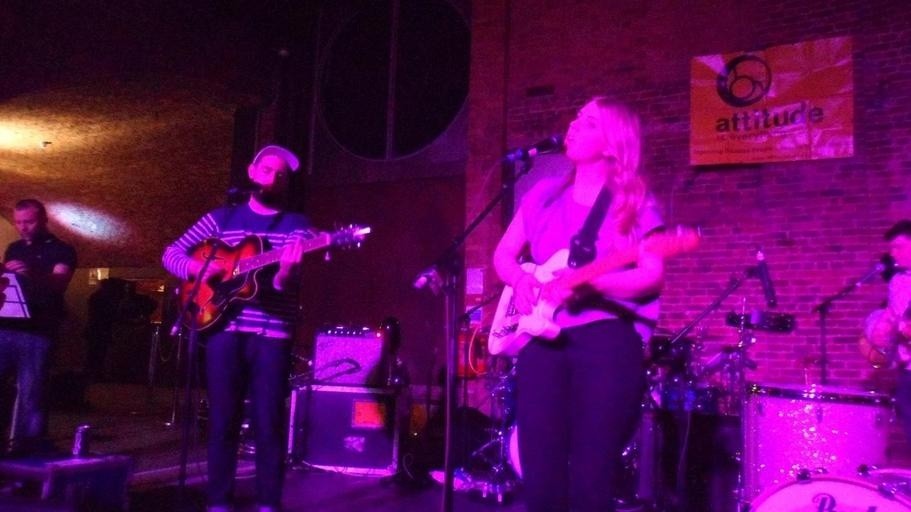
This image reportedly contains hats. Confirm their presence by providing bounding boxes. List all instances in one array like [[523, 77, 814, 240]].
[[251, 146, 301, 173]]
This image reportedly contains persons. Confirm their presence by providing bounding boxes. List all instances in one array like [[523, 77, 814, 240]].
[[490, 91, 667, 511], [857, 220, 911, 464], [0, 196, 77, 449], [160, 143, 321, 512]]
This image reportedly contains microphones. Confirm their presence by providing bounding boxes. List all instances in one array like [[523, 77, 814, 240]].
[[504, 134, 563, 163], [227, 184, 262, 200], [755, 247, 778, 307]]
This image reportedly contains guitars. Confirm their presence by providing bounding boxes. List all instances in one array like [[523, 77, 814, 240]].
[[178, 226, 374, 334], [862, 294, 909, 366], [489, 229, 692, 357]]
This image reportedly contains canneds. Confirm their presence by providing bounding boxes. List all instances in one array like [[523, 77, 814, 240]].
[[70, 424, 91, 456]]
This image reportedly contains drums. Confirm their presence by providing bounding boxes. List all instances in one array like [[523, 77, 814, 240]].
[[457, 327, 512, 383], [749, 466, 911, 510], [622, 406, 712, 507], [504, 423, 521, 477], [741, 379, 899, 509]]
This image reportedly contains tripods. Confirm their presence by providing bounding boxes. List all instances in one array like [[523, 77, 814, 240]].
[[455, 373, 516, 504]]
